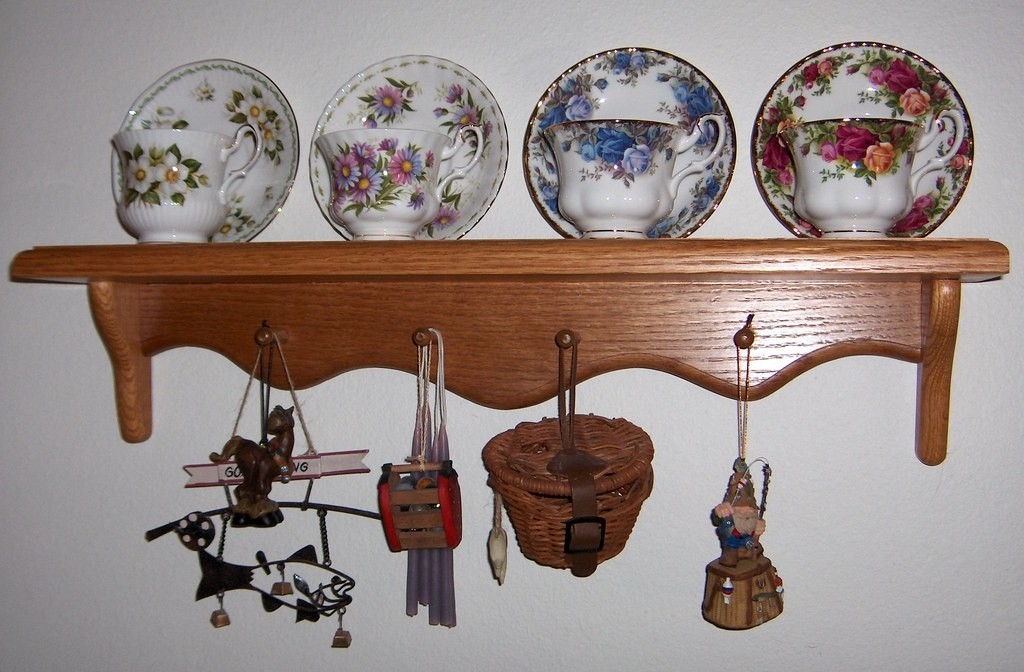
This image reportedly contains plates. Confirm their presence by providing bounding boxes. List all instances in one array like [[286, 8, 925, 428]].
[[308, 54, 509, 243], [748, 41, 973, 240], [522, 47, 736, 241], [110, 58, 300, 244]]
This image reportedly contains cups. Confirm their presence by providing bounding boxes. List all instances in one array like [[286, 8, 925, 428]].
[[314, 124, 483, 241], [544, 113, 726, 238], [110, 120, 262, 244], [779, 109, 964, 240]]
